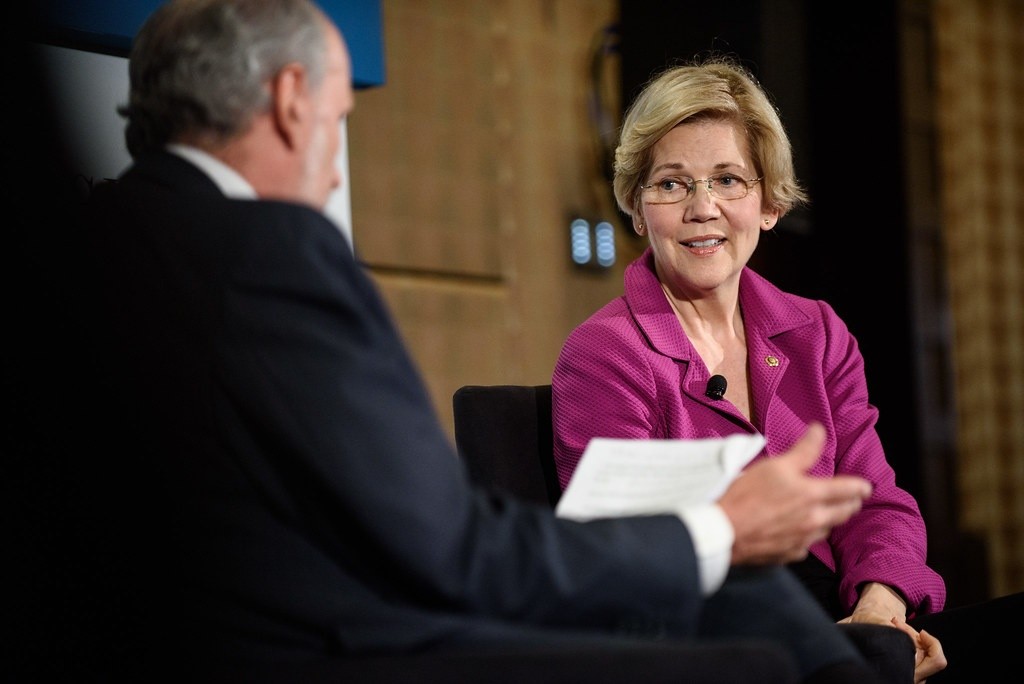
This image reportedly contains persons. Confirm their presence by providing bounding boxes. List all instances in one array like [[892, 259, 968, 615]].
[[0, 0, 874, 684], [549, 64, 950, 684]]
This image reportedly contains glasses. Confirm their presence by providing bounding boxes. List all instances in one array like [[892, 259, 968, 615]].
[[641, 173, 766, 205]]
